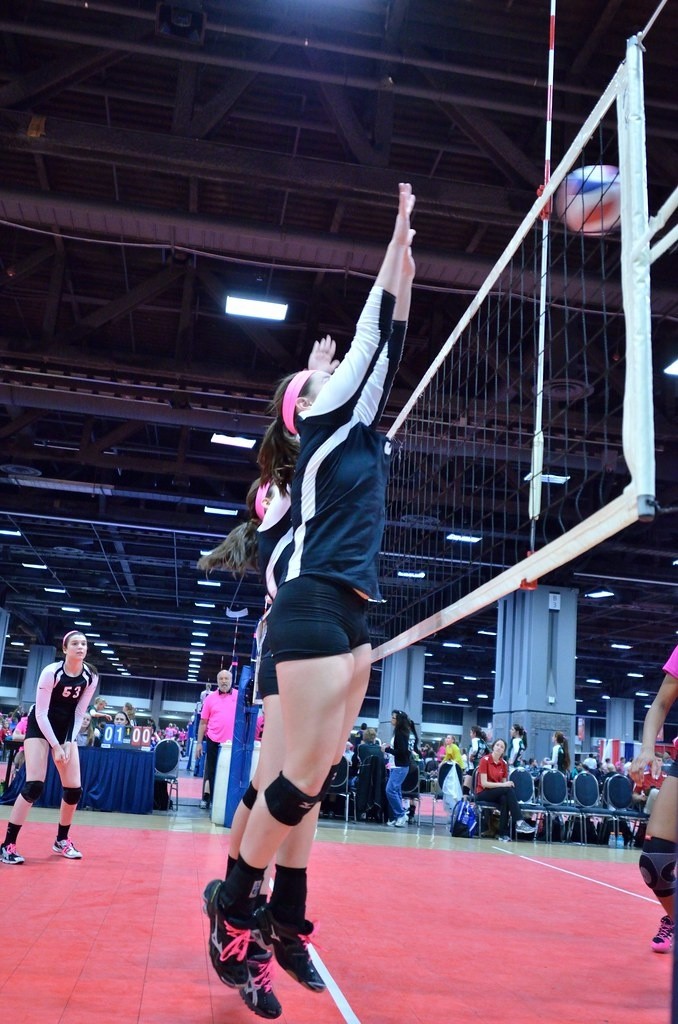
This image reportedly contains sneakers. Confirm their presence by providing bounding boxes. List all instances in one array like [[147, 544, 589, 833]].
[[205, 879, 251, 987], [396, 814, 409, 826], [499, 835, 512, 842], [515, 821, 535, 834], [53, 837, 83, 859], [238, 952, 283, 1020], [388, 820, 396, 827], [252, 905, 327, 993], [0, 843, 26, 865], [651, 915, 676, 952]]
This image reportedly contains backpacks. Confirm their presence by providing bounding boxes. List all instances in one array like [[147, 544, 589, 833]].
[[451, 797, 478, 836]]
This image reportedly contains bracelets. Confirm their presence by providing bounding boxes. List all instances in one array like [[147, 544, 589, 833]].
[[197, 741, 203, 745]]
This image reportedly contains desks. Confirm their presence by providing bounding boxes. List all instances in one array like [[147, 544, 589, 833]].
[[0, 746, 154, 815]]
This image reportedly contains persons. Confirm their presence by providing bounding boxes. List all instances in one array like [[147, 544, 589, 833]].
[[470, 723, 597, 842], [75, 697, 186, 756], [627, 643, 678, 952], [600, 751, 674, 801], [0, 630, 99, 862], [204, 181, 415, 1019], [194, 670, 264, 808], [343, 710, 462, 826], [0, 712, 28, 771]]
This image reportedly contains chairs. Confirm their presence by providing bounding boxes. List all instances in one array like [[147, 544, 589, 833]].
[[470, 766, 650, 845], [153, 738, 181, 811], [321, 749, 425, 826]]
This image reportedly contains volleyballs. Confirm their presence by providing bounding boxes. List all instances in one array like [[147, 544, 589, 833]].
[[556, 165, 621, 236]]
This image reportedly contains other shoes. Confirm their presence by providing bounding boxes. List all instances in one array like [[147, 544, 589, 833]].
[[199, 799, 209, 808]]
